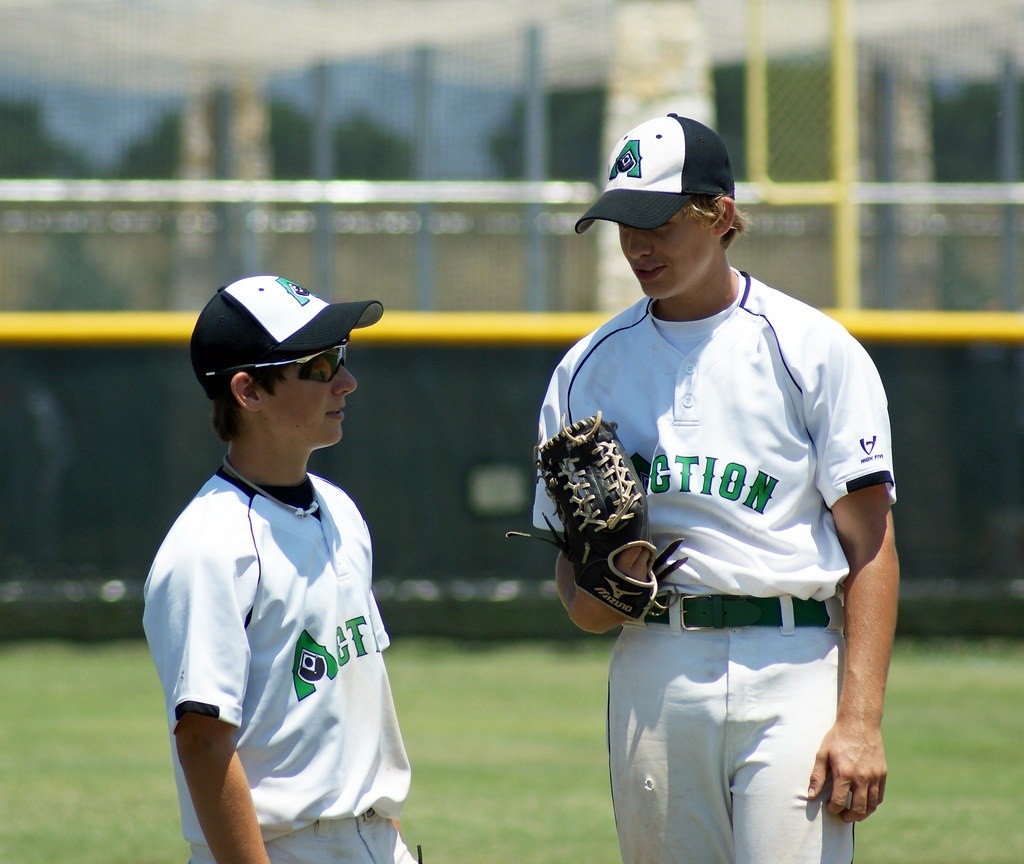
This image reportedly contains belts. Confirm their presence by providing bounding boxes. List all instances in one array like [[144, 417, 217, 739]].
[[644, 593, 830, 629]]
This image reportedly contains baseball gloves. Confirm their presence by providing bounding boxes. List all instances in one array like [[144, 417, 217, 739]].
[[536, 416, 659, 623]]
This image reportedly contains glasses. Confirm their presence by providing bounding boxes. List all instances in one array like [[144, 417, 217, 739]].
[[202, 340, 352, 381]]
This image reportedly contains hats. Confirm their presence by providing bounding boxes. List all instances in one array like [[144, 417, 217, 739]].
[[191, 274, 385, 399], [575, 112, 735, 235]]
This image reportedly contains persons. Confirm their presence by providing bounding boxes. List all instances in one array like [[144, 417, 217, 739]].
[[142, 277, 432, 864], [533, 112, 902, 864]]
